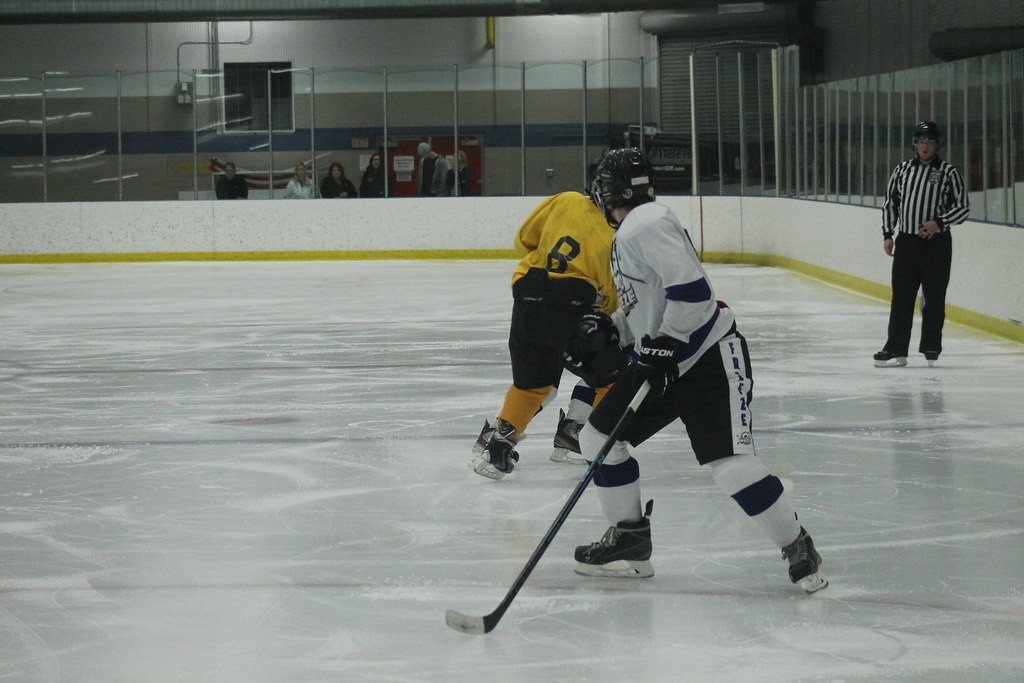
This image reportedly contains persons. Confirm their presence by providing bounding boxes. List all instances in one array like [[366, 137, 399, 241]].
[[445, 150, 470, 197], [575, 148, 823, 583], [873, 123, 971, 360], [283, 163, 322, 199], [479, 192, 621, 474], [215, 162, 249, 201], [321, 162, 358, 199], [361, 153, 395, 197], [417, 142, 448, 197]]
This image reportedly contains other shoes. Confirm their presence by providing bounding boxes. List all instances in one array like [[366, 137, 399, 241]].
[[873, 349, 908, 367], [924, 350, 938, 367]]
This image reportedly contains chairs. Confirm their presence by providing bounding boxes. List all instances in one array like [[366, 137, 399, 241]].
[[178, 188, 289, 200]]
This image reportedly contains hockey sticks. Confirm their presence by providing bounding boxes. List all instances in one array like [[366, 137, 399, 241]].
[[444, 375, 654, 636]]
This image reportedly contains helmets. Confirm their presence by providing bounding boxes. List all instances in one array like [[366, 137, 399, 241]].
[[911, 121, 942, 151], [593, 147, 654, 208]]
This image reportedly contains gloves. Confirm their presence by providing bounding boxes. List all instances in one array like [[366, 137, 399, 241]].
[[626, 334, 680, 405], [565, 312, 623, 386]]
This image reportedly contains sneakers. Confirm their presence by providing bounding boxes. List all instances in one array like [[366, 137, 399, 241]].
[[779, 512, 828, 593], [472, 419, 519, 466], [573, 499, 655, 578], [473, 416, 517, 479], [550, 408, 589, 464]]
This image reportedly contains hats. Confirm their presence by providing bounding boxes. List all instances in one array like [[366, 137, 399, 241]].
[[418, 142, 431, 155]]
